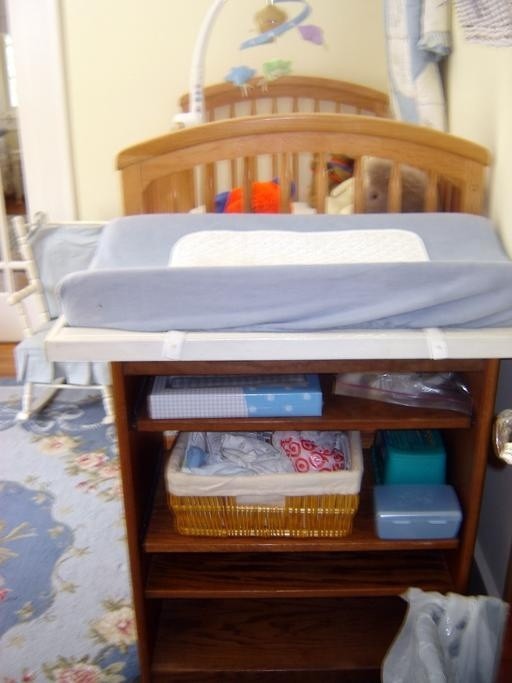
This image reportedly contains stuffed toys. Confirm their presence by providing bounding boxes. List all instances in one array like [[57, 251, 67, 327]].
[[308, 153, 444, 214], [215, 177, 296, 213]]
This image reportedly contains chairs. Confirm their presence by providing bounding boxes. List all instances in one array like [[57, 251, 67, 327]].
[[2, 215, 116, 426]]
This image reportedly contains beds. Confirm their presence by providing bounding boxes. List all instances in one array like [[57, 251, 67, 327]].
[[117, 71, 495, 217]]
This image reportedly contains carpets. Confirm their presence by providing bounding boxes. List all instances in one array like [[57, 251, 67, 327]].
[[2, 375, 512, 682]]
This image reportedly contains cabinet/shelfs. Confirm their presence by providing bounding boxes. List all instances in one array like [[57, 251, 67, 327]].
[[114, 359, 504, 682]]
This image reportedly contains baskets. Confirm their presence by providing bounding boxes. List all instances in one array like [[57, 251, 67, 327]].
[[163, 429, 366, 540]]
